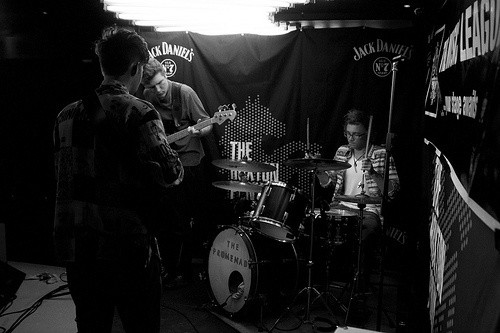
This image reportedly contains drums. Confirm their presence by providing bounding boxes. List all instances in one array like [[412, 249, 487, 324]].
[[328, 209, 358, 245], [208, 226, 300, 314], [252, 181, 310, 242]]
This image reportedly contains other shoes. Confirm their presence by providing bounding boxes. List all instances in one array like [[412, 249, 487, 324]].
[[165, 274, 194, 291]]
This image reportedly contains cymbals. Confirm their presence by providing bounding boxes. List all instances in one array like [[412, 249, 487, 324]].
[[284, 155, 352, 172], [333, 193, 390, 205], [212, 179, 266, 192], [212, 158, 278, 172]]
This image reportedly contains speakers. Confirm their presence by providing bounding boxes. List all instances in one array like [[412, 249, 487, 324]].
[[0, 261, 26, 309]]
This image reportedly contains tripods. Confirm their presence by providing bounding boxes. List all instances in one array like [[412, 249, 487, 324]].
[[267, 166, 340, 333]]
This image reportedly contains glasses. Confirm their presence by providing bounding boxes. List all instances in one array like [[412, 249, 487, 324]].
[[343, 130, 367, 140]]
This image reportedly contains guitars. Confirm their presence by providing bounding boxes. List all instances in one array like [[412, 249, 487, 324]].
[[167, 103, 237, 145]]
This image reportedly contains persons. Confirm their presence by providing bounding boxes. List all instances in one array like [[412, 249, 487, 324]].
[[136, 56, 212, 292], [52, 21, 184, 333], [305, 109, 400, 314]]
